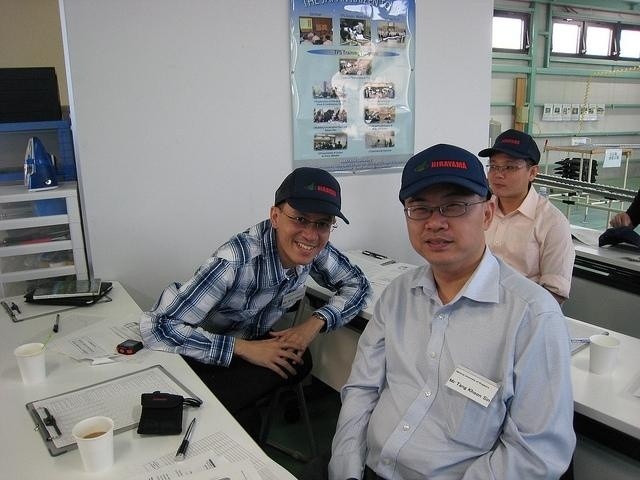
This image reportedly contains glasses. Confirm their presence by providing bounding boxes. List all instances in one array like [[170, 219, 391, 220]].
[[403, 201, 486, 220], [485, 163, 530, 173], [279, 207, 333, 233]]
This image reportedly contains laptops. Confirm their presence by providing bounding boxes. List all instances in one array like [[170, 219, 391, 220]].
[[22, 283, 114, 305]]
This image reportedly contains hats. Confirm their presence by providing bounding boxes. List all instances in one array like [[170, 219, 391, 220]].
[[274, 166, 350, 226], [398, 142, 489, 200], [478, 128, 541, 167]]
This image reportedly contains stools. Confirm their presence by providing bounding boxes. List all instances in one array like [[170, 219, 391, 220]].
[[234, 332, 323, 480]]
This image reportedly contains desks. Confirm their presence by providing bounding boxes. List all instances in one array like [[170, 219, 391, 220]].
[[544, 145, 633, 228], [299, 248, 639, 480], [559, 224, 639, 339], [0, 280, 295, 480]]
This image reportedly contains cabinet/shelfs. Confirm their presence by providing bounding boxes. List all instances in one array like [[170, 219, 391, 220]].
[[1, 121, 93, 300]]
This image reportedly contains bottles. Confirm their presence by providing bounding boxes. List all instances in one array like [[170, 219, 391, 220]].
[[539, 186, 546, 197]]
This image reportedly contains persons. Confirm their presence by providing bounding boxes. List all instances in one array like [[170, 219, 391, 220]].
[[327, 144, 578, 480], [608, 185, 640, 230], [138, 167, 371, 411], [479, 128, 576, 306]]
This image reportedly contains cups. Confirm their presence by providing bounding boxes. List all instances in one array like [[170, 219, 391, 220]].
[[72, 416, 114, 474], [14, 342, 47, 386], [588, 335, 621, 377]]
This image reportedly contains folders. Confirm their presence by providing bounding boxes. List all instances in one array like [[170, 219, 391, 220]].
[[26, 364, 203, 458], [0, 294, 113, 323]]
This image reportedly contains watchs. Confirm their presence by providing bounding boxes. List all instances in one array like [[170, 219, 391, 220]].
[[312, 312, 327, 331]]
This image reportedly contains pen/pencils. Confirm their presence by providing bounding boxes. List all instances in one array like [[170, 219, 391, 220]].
[[53, 314, 60, 332], [571, 339, 591, 343], [175, 418, 196, 461]]
